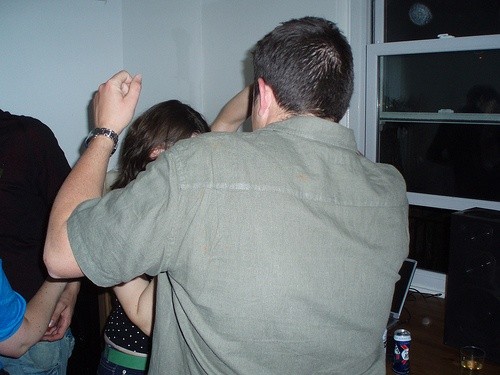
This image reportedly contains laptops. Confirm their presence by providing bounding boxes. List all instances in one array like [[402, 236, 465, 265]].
[[387, 258, 418, 326]]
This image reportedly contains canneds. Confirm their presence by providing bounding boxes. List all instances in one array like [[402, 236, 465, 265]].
[[392, 329, 411, 374]]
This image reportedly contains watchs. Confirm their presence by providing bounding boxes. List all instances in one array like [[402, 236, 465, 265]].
[[84, 128, 119, 158]]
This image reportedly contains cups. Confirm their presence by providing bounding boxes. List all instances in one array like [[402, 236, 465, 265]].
[[459, 346, 486, 375]]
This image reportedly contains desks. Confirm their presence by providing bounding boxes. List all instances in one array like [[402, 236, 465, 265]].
[[386, 290, 500, 375]]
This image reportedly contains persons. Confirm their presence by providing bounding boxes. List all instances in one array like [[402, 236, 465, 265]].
[[0, 99, 212, 375], [42, 17, 409, 375]]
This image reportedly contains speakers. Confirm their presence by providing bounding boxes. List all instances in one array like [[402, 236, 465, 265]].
[[443, 207, 500, 366]]
